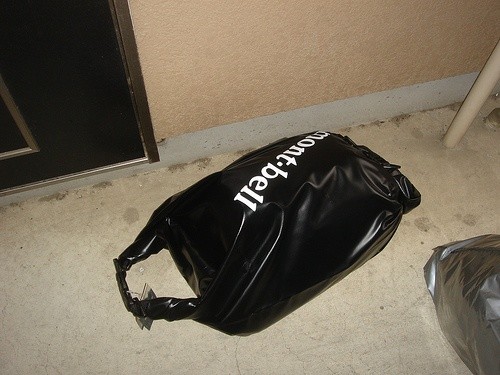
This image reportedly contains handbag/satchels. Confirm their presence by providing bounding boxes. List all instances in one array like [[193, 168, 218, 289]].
[[114, 131, 422, 336], [423, 234, 499, 375]]
